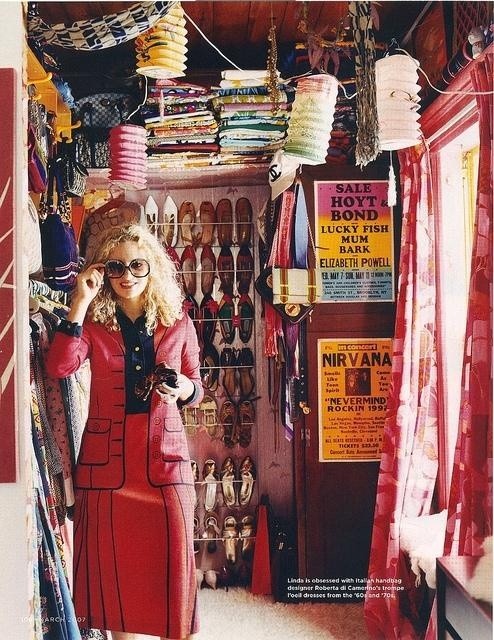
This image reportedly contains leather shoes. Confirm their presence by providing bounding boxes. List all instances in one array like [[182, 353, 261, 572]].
[[201, 245, 216, 294], [181, 294, 254, 344], [218, 248, 234, 295], [163, 196, 178, 247], [144, 195, 158, 240], [236, 247, 253, 294], [180, 245, 196, 296]]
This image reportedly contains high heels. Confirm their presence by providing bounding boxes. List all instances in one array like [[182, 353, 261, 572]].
[[205, 570, 217, 590], [239, 565, 249, 589], [196, 568, 203, 590], [222, 567, 233, 592]]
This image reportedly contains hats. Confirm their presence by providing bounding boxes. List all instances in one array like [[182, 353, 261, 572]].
[[268, 149, 301, 202]]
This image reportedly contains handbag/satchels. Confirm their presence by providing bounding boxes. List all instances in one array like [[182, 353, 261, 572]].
[[271, 181, 323, 307], [72, 128, 111, 168], [271, 518, 297, 604], [73, 89, 140, 129], [254, 181, 317, 325], [27, 84, 88, 291]]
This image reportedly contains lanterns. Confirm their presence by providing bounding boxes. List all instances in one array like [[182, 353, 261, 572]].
[[373, 54, 421, 153], [108, 123, 149, 192], [133, 1, 188, 80], [280, 73, 340, 165]]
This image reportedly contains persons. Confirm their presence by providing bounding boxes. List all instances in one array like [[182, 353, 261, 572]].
[[44, 220, 206, 640]]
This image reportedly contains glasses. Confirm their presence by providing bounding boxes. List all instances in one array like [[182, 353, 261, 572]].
[[134, 361, 177, 407], [105, 258, 150, 279]]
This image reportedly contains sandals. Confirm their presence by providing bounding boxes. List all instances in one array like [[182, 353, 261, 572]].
[[190, 455, 256, 565], [216, 199, 232, 247], [197, 201, 216, 248], [181, 344, 255, 448], [179, 200, 199, 247], [235, 198, 252, 247]]
